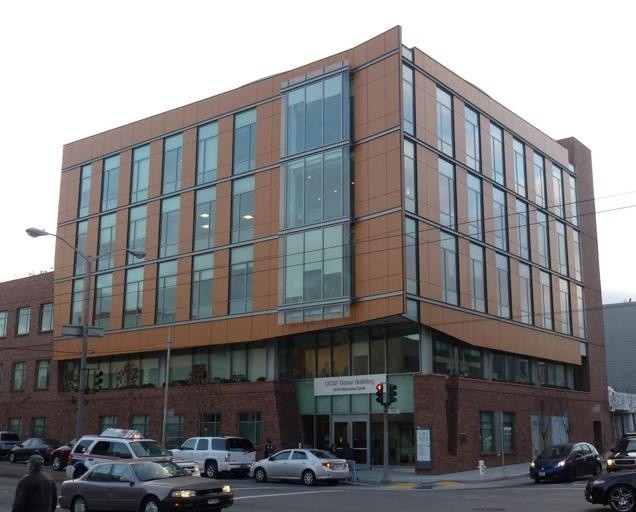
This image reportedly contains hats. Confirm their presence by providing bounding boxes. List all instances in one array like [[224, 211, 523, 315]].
[[25, 455, 44, 464]]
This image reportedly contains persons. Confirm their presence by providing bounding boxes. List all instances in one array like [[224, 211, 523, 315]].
[[341, 442, 357, 481], [263, 438, 273, 457], [11, 455, 58, 512], [329, 442, 339, 457]]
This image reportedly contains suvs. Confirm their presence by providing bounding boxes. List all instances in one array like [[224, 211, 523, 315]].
[[64, 428, 201, 477], [605, 432, 636, 472], [168, 434, 258, 478]]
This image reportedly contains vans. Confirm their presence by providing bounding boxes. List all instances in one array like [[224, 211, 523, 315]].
[[530, 441, 603, 484]]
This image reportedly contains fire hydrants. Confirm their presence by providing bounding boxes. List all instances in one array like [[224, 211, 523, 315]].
[[475, 458, 488, 478]]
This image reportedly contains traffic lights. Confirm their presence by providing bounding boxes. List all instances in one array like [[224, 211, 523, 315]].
[[375, 383, 383, 404], [388, 383, 398, 404]]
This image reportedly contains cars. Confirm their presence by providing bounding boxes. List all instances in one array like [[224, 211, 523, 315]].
[[0, 430, 81, 471], [584, 469, 636, 512], [56, 459, 234, 512], [249, 448, 351, 486]]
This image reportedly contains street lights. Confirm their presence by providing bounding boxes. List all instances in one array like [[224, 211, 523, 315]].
[[23, 228, 146, 437]]
